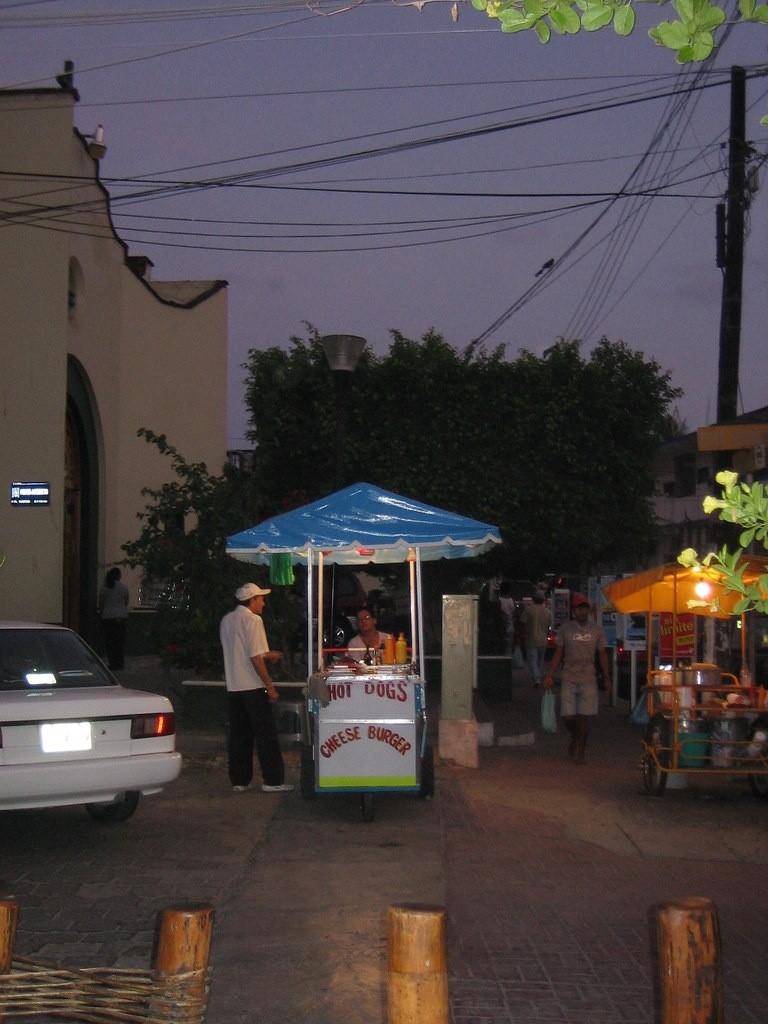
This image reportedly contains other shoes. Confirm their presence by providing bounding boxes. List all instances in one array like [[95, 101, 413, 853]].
[[569, 740, 577, 754], [576, 752, 588, 764], [533, 681, 540, 689], [233, 784, 252, 793], [263, 784, 294, 792]]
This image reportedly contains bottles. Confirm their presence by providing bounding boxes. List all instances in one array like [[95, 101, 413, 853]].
[[384, 634, 396, 663], [395, 632, 407, 664], [364, 644, 372, 665]]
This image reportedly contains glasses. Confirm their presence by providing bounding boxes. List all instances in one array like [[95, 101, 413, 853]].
[[358, 616, 369, 622]]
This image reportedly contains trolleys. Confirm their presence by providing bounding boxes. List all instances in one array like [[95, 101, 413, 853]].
[[225, 479, 501, 824], [601, 553, 768, 799]]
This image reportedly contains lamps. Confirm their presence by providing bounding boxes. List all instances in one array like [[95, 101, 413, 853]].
[[80, 125, 107, 159]]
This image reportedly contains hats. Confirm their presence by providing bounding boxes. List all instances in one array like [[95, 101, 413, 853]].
[[235, 583, 271, 601], [535, 590, 545, 601], [571, 593, 590, 608]]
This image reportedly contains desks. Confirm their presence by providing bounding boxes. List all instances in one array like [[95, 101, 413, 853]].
[[181, 679, 306, 688], [424, 655, 513, 702]]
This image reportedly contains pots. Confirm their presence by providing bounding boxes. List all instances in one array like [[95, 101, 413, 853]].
[[681, 668, 724, 687]]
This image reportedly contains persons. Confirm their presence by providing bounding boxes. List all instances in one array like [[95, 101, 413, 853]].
[[220, 583, 296, 792], [99, 567, 130, 671], [495, 592, 553, 684], [346, 609, 396, 666], [543, 594, 611, 765]]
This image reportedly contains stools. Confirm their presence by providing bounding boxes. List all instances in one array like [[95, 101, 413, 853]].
[[274, 698, 310, 750]]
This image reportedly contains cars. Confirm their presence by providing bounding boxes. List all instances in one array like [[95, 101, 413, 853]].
[[0, 618, 183, 825], [510, 575, 648, 676]]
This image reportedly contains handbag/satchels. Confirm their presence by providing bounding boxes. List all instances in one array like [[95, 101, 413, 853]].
[[512, 644, 524, 669], [541, 688, 558, 735]]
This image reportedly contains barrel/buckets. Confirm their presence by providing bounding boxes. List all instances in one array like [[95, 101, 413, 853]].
[[678, 732, 709, 768]]
